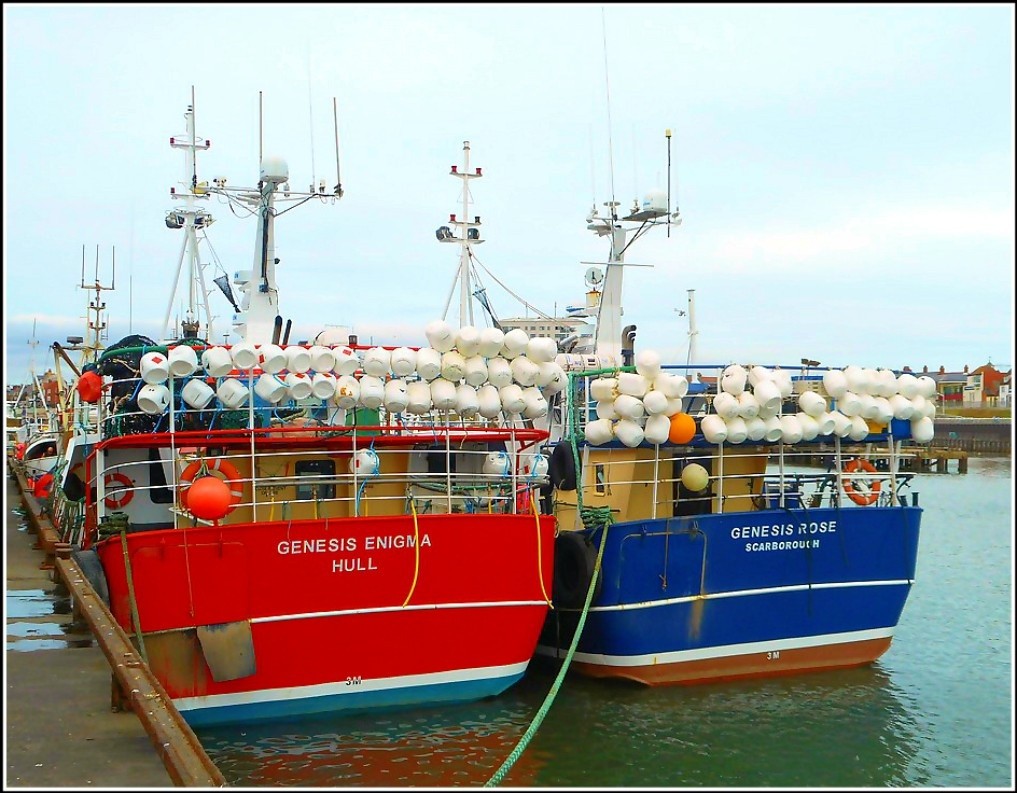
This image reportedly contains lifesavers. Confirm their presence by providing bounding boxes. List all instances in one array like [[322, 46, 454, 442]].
[[517, 485, 529, 515], [542, 441, 582, 495], [554, 530, 603, 607], [177, 459, 244, 520], [842, 459, 881, 505], [104, 473, 134, 509]]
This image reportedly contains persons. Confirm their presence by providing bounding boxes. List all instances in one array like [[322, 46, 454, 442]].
[[41, 446, 53, 457]]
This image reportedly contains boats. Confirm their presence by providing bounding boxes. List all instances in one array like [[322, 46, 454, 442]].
[[427, 128, 942, 688], [14, 84, 579, 727]]
